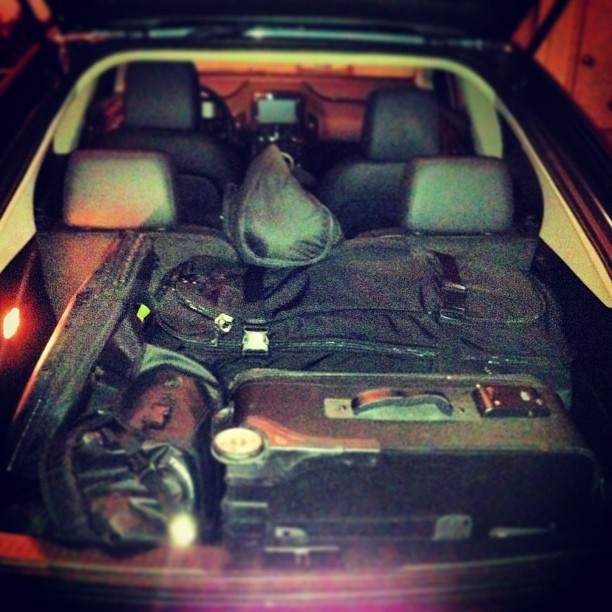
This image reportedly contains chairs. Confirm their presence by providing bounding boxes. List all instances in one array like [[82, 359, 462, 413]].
[[33, 149, 178, 306], [327, 85, 449, 236], [107, 58, 239, 226], [348, 155, 543, 280]]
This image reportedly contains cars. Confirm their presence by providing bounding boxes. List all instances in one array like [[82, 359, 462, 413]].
[[2, 0, 610, 606]]
